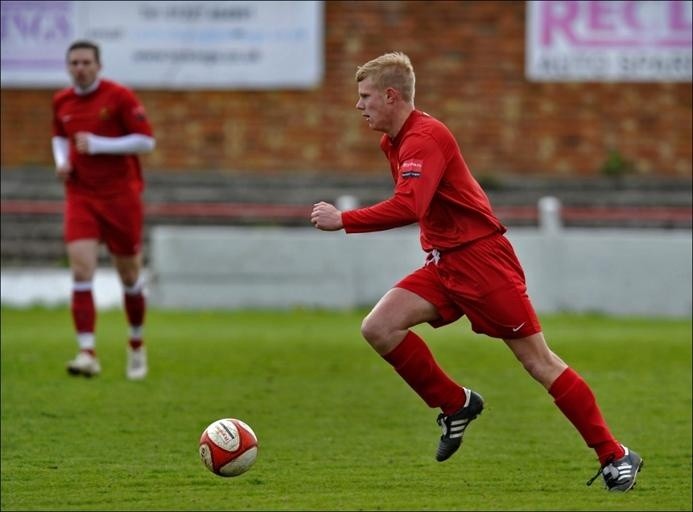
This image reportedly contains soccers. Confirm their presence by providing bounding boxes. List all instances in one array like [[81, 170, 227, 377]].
[[198, 417, 260, 478]]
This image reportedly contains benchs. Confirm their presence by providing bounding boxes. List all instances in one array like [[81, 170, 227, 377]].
[[1, 164, 693, 271]]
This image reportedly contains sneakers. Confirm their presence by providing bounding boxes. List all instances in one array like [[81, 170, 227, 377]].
[[67, 351, 100, 378], [127, 344, 147, 380], [587, 445, 644, 493], [435, 387, 484, 461]]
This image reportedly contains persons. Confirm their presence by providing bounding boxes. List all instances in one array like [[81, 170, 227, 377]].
[[45, 41, 159, 381], [308, 52, 646, 494]]
